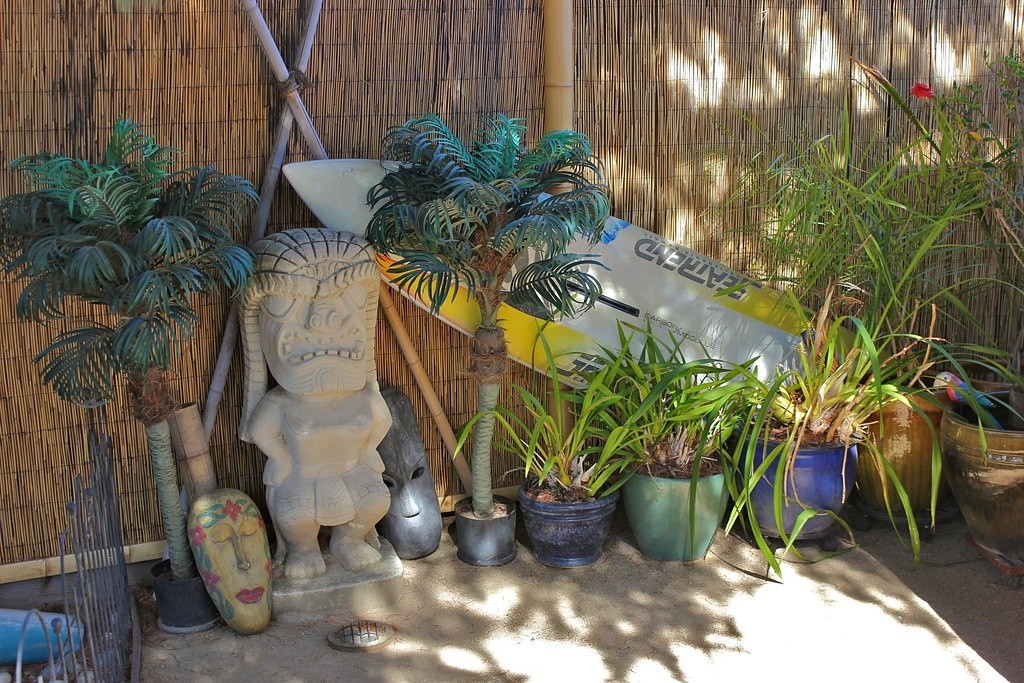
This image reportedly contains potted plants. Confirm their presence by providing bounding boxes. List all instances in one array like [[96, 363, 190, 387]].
[[364, 49, 1024, 589], [0, 114, 261, 633]]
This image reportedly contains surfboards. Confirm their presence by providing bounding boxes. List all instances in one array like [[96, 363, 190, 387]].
[[280, 158, 908, 425]]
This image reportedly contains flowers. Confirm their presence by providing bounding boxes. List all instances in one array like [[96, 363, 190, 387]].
[[910, 82, 934, 99]]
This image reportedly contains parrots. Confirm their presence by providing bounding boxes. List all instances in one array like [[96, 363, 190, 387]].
[[933, 372, 1002, 429]]
